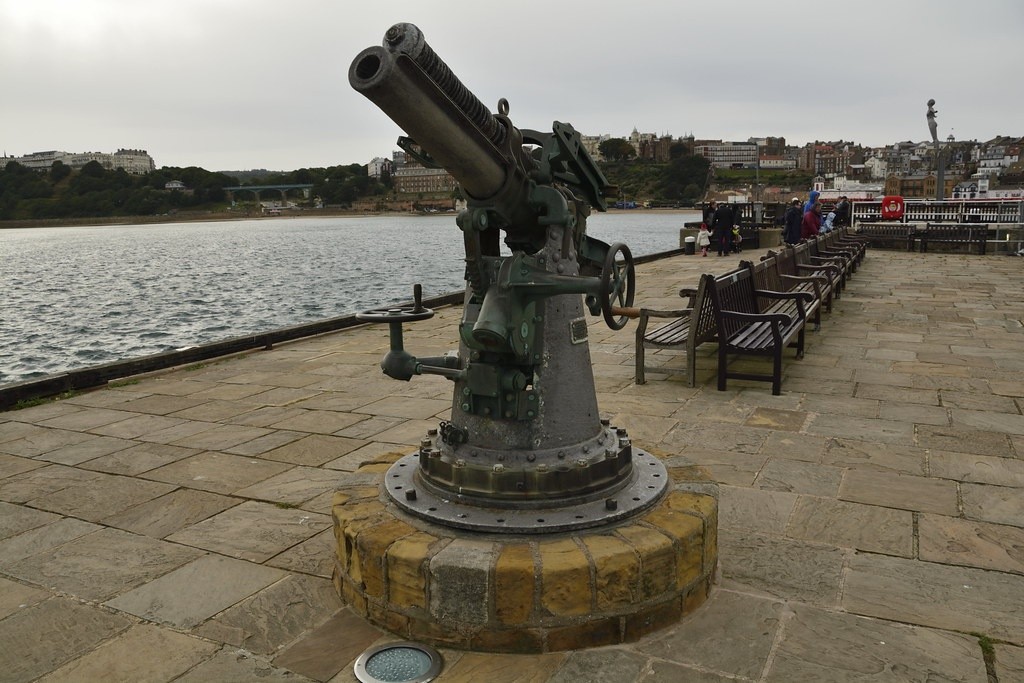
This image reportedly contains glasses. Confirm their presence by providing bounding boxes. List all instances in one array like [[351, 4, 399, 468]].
[[799, 205, 802, 207]]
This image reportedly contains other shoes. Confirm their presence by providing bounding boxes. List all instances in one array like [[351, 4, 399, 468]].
[[717, 254, 721, 257], [702, 254, 707, 257], [724, 254, 730, 256]]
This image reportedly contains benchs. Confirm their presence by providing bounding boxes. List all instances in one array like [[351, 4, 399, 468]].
[[635, 223, 989, 396]]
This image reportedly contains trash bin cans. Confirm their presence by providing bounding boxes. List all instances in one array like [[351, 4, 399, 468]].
[[759, 228, 783, 247]]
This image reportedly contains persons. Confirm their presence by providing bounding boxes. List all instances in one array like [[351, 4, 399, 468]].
[[696, 201, 743, 257], [926, 99, 938, 141], [782, 192, 850, 248]]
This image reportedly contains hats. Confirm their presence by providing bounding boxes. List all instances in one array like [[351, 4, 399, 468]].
[[700, 223, 708, 230], [841, 196, 847, 199]]
